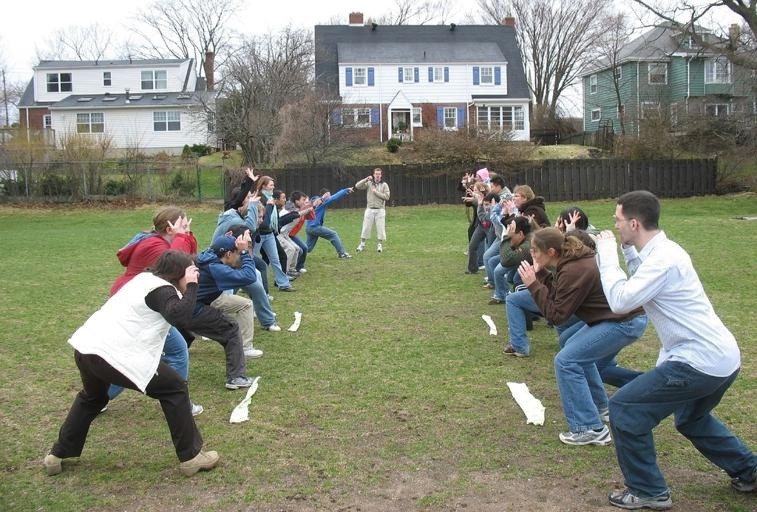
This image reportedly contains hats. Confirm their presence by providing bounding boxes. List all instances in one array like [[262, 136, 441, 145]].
[[213, 236, 236, 251]]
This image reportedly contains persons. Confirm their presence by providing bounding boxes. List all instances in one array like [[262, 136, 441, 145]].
[[456, 165, 646, 445], [97, 165, 355, 421], [37, 248, 220, 477], [586, 188, 756, 511], [355, 162, 390, 255]]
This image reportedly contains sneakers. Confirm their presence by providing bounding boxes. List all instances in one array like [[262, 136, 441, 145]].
[[226, 375, 253, 389], [244, 349, 263, 359], [503, 346, 527, 357], [338, 253, 352, 258], [357, 243, 382, 252], [559, 410, 612, 446], [180, 449, 219, 476], [609, 489, 672, 510], [45, 455, 62, 476], [275, 269, 307, 291], [466, 265, 502, 304], [731, 468, 757, 491], [191, 404, 203, 416], [269, 325, 281, 332]]
